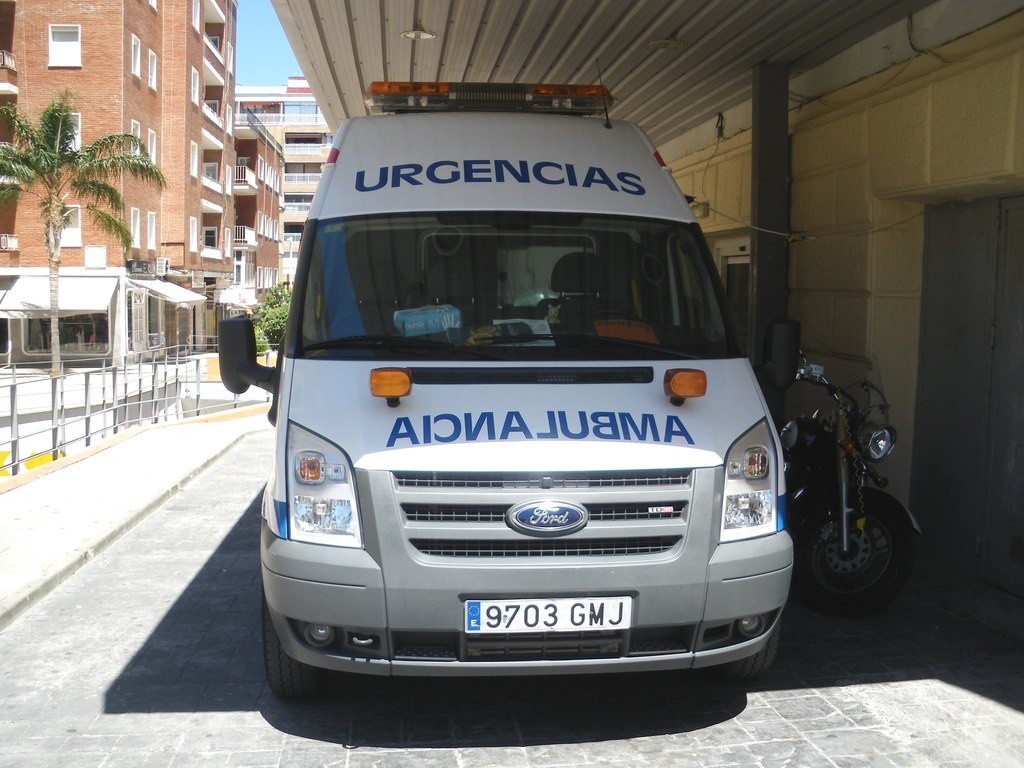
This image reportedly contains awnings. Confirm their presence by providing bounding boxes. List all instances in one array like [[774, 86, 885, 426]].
[[0, 276, 118, 311], [131, 278, 207, 302]]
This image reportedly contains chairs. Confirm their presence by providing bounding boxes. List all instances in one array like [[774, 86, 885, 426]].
[[536, 252, 618, 332]]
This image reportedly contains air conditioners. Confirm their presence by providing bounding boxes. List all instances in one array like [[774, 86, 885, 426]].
[[0, 52, 16, 69], [156, 256, 170, 275], [1, 236, 18, 249]]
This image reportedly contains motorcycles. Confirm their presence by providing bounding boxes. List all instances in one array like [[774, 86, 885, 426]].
[[759, 314, 927, 616]]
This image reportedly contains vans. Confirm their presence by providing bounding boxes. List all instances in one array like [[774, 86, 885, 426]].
[[215, 80, 796, 706]]
